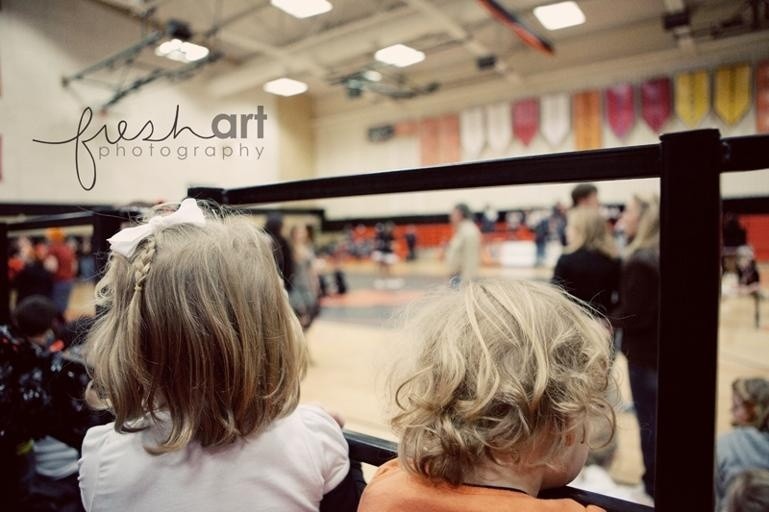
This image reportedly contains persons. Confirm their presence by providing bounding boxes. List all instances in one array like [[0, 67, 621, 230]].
[[0, 180, 768, 332], [603, 188, 659, 498], [346, 275, 615, 512], [0, 293, 114, 512], [719, 467, 768, 510], [713, 372, 768, 507], [66, 194, 366, 512], [549, 206, 624, 383]]
